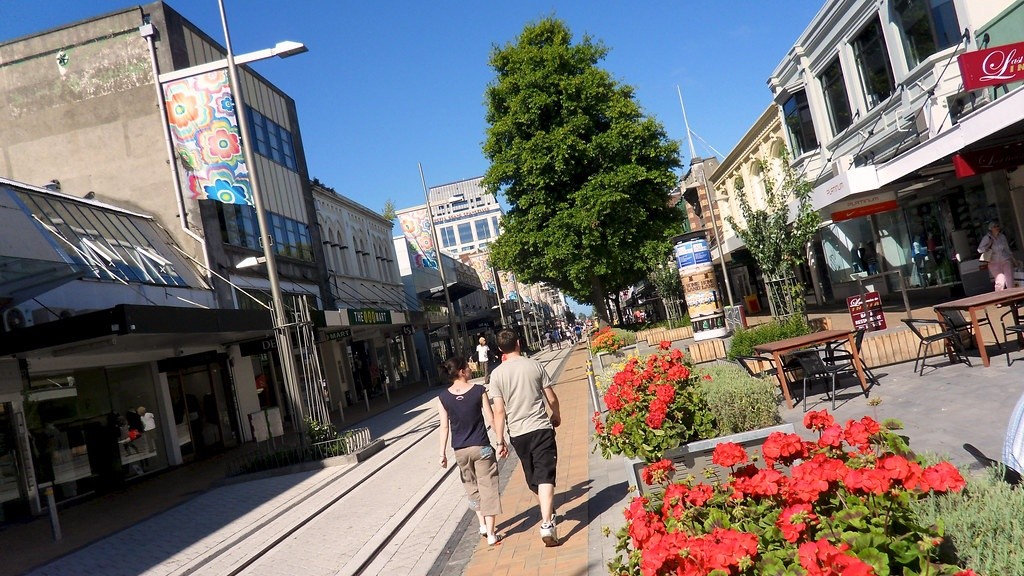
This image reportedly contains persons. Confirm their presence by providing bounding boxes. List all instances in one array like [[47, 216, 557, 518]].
[[117, 416, 139, 457], [437, 357, 509, 546], [138, 407, 156, 453], [545, 328, 562, 352], [565, 324, 581, 343], [851, 239, 877, 274], [912, 233, 939, 286], [490, 328, 562, 547], [594, 317, 598, 328], [635, 309, 646, 322], [978, 221, 1018, 307], [472, 337, 498, 384]]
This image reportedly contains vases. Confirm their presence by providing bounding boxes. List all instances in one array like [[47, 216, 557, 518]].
[[595, 343, 639, 369], [623, 415, 802, 507]]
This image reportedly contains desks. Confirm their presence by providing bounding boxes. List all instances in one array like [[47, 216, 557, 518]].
[[752, 329, 870, 410], [933, 285, 1024, 367]]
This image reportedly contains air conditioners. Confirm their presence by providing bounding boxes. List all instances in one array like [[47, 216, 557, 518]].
[[32, 307, 74, 326], [1, 305, 34, 330]]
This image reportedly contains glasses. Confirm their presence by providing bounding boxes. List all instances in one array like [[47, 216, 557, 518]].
[[992, 225, 1000, 228]]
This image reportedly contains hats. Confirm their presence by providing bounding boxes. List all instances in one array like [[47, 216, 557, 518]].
[[565, 326, 569, 329]]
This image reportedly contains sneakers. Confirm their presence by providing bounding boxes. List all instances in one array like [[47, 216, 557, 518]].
[[540, 522, 559, 547], [550, 514, 556, 527]]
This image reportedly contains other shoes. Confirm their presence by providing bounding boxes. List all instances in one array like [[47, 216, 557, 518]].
[[148, 448, 154, 453], [996, 304, 1001, 307], [479, 525, 489, 535], [487, 535, 503, 544], [125, 453, 132, 457], [134, 452, 139, 454]]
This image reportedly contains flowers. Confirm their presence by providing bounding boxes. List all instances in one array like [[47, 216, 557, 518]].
[[601, 411, 1024, 576], [583, 326, 782, 459]]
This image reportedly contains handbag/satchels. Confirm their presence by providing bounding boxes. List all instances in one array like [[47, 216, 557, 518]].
[[909, 262, 921, 287], [128, 429, 141, 441], [979, 249, 992, 262]]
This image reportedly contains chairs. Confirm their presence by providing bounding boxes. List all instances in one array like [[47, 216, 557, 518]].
[[732, 326, 880, 412], [900, 308, 1002, 377], [1000, 303, 1024, 366]]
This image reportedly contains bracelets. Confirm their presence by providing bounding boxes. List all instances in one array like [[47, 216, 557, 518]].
[[440, 456, 446, 458]]
